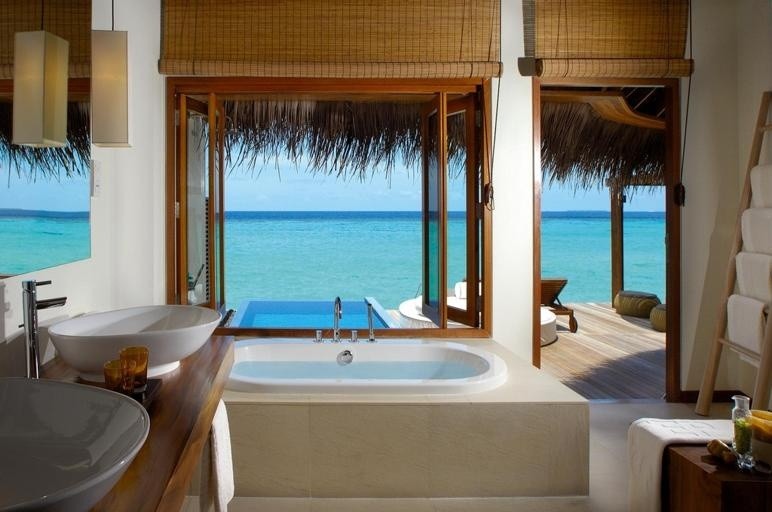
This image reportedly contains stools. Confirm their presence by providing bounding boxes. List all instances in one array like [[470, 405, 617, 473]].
[[614, 290, 661, 318], [650, 303, 667, 332]]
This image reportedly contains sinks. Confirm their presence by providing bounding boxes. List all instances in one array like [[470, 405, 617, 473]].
[[0, 376, 150, 512], [47, 304, 222, 383]]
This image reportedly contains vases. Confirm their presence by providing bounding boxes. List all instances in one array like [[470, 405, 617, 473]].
[[732, 395, 752, 459]]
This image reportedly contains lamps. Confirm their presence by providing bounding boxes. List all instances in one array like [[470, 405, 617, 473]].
[[89, 0, 134, 149], [12, 0, 70, 148]]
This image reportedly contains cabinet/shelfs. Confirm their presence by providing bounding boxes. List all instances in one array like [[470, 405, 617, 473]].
[[630, 418, 772, 512]]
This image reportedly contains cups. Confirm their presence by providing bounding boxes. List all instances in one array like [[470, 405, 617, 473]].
[[103, 358, 136, 398], [119, 344, 150, 393]]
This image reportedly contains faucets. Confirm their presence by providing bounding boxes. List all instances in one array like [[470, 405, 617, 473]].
[[14, 278, 67, 378], [333, 296, 342, 341]]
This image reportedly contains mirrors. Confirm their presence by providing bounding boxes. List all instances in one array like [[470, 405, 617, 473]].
[[0, 0, 92, 280]]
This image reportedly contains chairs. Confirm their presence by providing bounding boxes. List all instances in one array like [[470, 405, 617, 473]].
[[540, 279, 577, 333]]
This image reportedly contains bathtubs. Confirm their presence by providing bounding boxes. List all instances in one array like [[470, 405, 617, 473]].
[[223, 337, 508, 392]]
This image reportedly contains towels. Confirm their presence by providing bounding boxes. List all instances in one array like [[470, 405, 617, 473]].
[[200, 397, 235, 512], [726, 163, 772, 369], [626, 418, 734, 512]]
